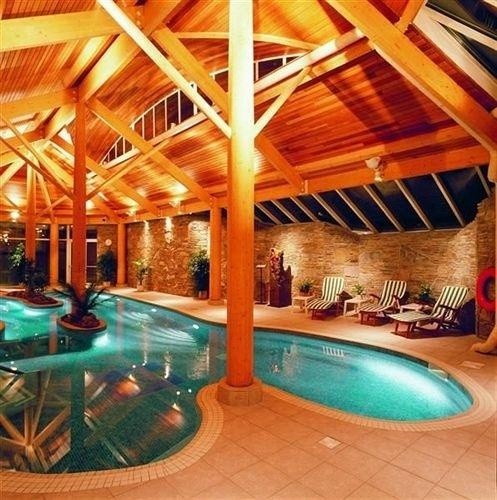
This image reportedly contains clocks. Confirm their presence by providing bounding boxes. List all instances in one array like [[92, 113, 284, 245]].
[[105, 238, 112, 246]]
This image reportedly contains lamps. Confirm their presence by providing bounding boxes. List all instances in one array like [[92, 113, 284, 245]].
[[365, 156, 384, 182]]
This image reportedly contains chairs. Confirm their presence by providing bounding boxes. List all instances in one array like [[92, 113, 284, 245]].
[[359, 280, 407, 326], [386, 286, 470, 337], [306, 277, 345, 319]]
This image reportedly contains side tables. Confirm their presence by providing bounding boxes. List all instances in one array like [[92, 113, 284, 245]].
[[343, 298, 368, 316], [292, 296, 314, 313], [400, 302, 429, 314]]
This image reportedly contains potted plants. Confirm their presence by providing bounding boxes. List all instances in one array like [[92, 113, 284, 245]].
[[416, 286, 434, 305], [352, 283, 365, 299], [188, 247, 210, 300], [293, 277, 315, 297], [97, 248, 116, 288], [132, 256, 152, 292]]
